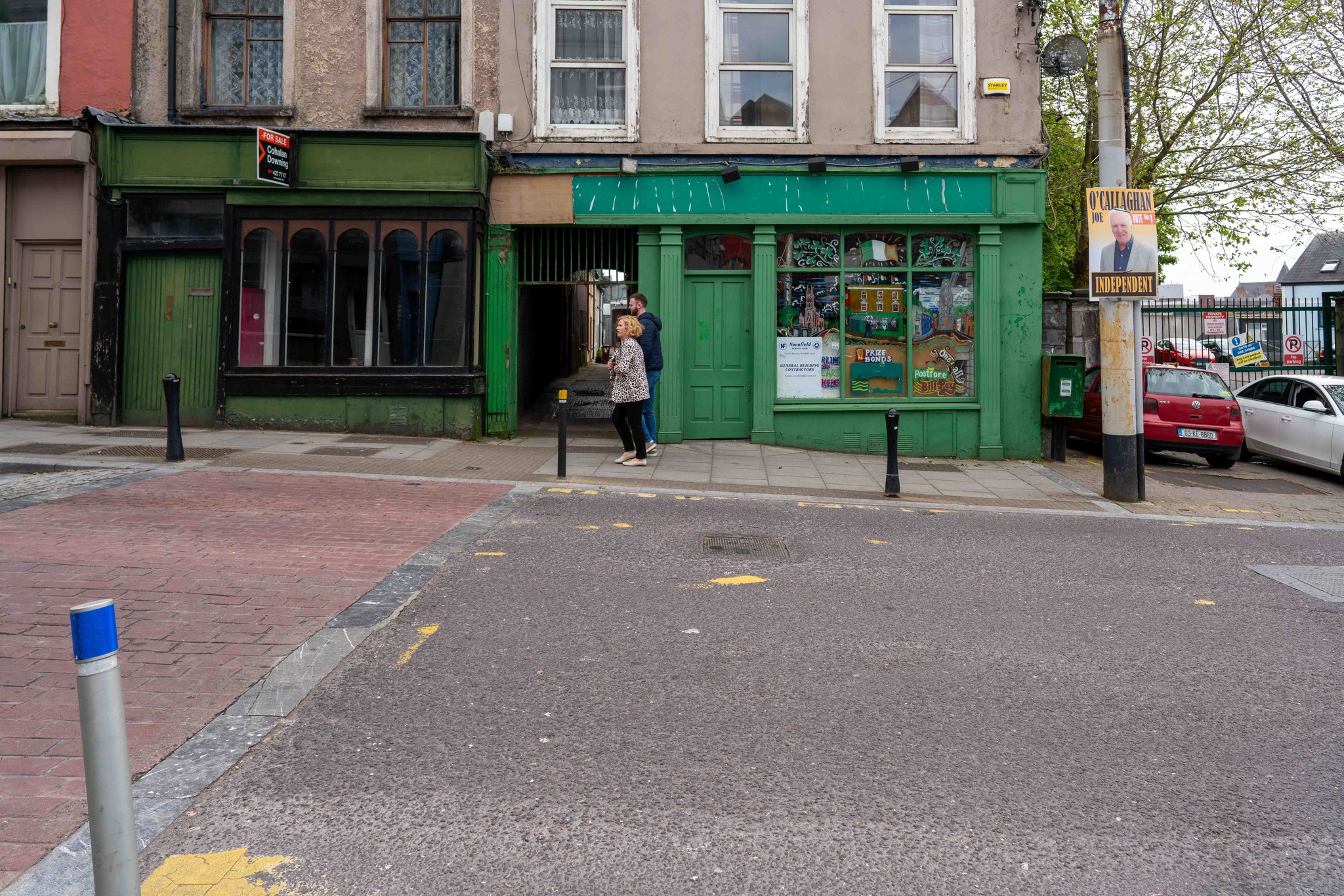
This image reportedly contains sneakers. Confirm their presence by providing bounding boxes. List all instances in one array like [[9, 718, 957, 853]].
[[646, 439, 656, 453], [646, 448, 657, 457]]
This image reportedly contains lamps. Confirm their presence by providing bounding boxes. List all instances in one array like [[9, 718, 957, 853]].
[[620, 155, 638, 174], [721, 161, 741, 183], [808, 157, 826, 172], [901, 156, 919, 172]]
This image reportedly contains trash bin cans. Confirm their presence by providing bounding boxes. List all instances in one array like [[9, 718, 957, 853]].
[[601, 345, 610, 364]]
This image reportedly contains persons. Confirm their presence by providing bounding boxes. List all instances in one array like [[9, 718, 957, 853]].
[[1100, 208, 1158, 300], [607, 315, 650, 466], [628, 293, 663, 456]]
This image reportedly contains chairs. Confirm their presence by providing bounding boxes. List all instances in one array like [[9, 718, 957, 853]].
[[1296, 387, 1317, 408], [1147, 378, 1204, 397]]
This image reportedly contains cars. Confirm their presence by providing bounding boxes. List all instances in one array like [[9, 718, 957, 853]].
[[1198, 339, 1268, 367], [1231, 374, 1344, 484], [1065, 361, 1245, 469], [1154, 338, 1215, 368]]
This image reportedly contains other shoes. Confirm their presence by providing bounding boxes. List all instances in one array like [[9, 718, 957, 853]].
[[623, 459, 647, 466], [614, 451, 637, 462]]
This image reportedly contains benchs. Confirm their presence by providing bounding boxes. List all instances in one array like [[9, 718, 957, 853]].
[[1260, 391, 1284, 403]]
